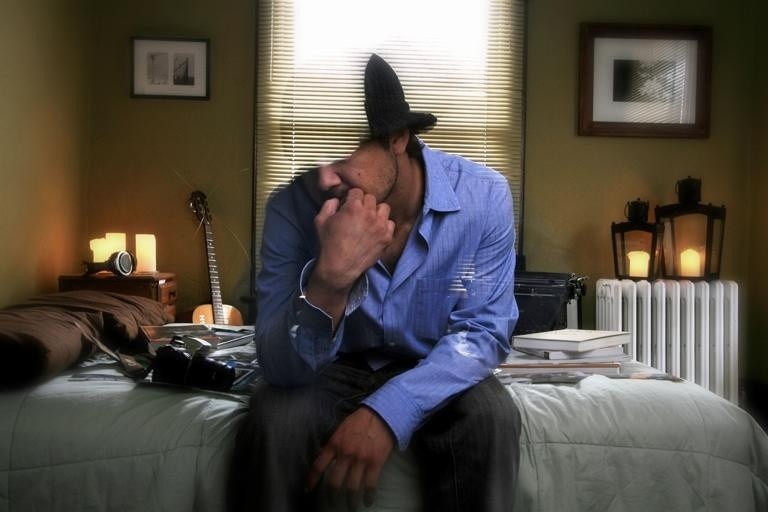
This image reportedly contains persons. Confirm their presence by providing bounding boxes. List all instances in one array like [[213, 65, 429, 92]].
[[223, 51, 522, 511]]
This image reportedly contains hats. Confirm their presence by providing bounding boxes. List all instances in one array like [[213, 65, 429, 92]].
[[277, 47, 435, 155]]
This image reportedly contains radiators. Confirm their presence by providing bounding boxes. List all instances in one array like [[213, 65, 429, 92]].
[[595, 278, 739, 407]]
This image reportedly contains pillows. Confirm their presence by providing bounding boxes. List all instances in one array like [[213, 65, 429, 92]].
[[25, 290, 176, 354], [0, 303, 106, 392]]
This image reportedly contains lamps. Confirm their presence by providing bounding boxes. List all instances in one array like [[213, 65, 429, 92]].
[[655, 175, 726, 281], [611, 198, 658, 280]]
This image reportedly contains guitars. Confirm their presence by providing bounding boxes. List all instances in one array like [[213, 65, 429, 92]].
[[188, 190, 243, 326]]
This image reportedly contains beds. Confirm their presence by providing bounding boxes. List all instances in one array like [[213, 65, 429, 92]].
[[0, 349, 768, 512]]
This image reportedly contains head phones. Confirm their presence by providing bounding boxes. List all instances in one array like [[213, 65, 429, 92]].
[[83, 250, 137, 276]]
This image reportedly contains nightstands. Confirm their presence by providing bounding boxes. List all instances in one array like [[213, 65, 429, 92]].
[[59, 270, 178, 316]]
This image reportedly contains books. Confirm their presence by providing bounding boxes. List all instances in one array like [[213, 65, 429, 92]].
[[498, 328, 632, 383], [138, 323, 265, 392]]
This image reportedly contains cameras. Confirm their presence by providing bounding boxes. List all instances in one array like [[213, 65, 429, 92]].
[[151, 336, 235, 392]]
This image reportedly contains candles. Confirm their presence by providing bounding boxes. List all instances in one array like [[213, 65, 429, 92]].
[[106, 232, 126, 253], [135, 234, 157, 273], [89, 238, 114, 273]]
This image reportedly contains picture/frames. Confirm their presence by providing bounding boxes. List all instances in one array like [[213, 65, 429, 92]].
[[578, 22, 714, 139], [129, 36, 210, 101]]
